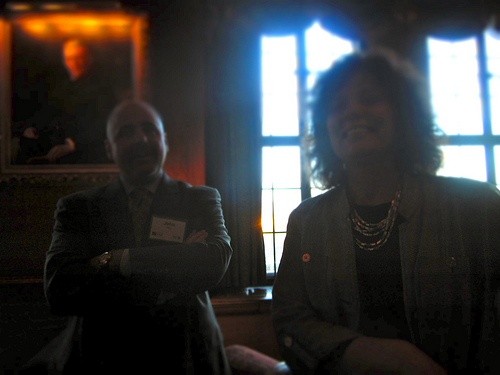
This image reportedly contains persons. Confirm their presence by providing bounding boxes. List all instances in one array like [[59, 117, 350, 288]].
[[272, 47, 500, 375], [45, 98, 233, 375], [19, 40, 121, 164]]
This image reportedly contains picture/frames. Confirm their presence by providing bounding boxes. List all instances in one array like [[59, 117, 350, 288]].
[[0, 11, 149, 183]]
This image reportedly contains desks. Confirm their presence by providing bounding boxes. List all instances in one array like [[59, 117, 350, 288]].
[[210, 284, 280, 360]]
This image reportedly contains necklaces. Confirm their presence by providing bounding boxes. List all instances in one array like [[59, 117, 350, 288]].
[[350, 191, 401, 252]]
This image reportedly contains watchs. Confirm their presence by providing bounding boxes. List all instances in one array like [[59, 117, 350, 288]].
[[97, 252, 111, 273]]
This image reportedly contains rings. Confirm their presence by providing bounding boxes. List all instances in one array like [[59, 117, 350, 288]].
[[415, 370, 425, 375]]
